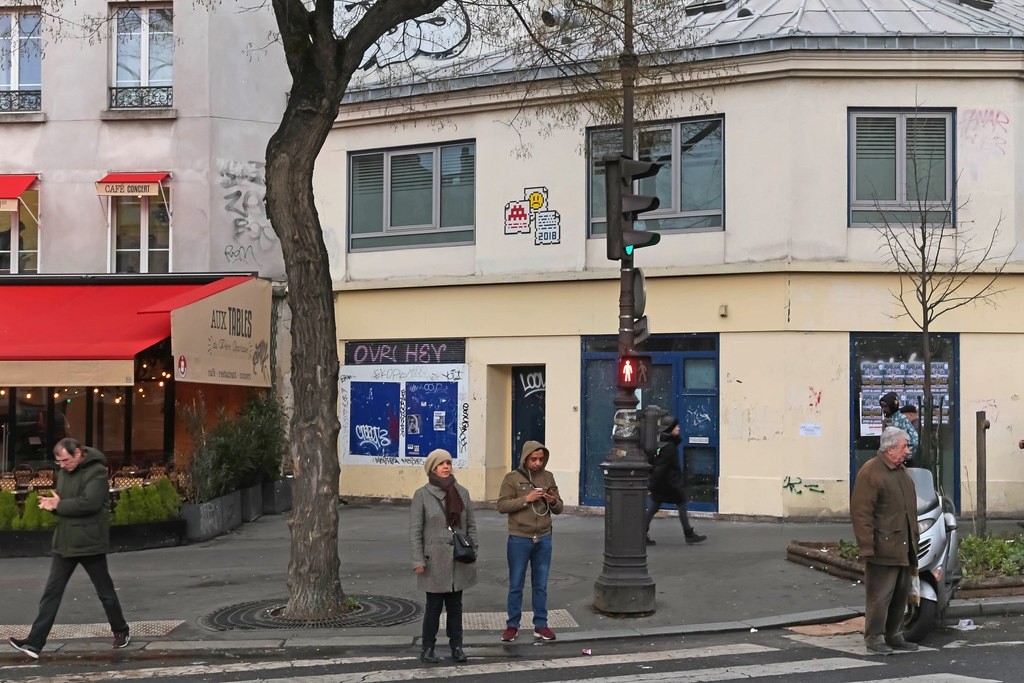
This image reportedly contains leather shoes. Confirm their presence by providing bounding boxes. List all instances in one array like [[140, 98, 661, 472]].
[[452, 647, 468, 661], [420, 648, 437, 662]]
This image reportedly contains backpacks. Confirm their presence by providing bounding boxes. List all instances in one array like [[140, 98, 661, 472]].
[[647, 449, 668, 492]]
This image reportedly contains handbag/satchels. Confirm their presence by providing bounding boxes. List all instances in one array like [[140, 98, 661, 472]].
[[909, 575, 922, 607], [452, 534, 477, 563]]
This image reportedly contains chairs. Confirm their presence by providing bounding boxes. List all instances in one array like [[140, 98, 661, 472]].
[[0, 462, 193, 512]]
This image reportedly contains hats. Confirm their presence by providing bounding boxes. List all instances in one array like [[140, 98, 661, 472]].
[[879, 392, 900, 413], [900, 405, 916, 413], [658, 416, 679, 433], [425, 449, 452, 475]]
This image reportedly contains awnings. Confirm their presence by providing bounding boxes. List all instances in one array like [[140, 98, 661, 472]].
[[0, 175, 39, 224], [0, 284, 207, 387], [96, 172, 169, 224]]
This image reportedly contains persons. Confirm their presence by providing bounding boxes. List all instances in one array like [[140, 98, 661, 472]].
[[879, 392, 919, 466], [644, 416, 707, 545], [901, 406, 930, 468], [496, 439, 564, 640], [8, 438, 130, 660], [852, 426, 921, 653], [408, 449, 478, 663]]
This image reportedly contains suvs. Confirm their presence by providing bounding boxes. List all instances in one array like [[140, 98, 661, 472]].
[[0, 407, 72, 459]]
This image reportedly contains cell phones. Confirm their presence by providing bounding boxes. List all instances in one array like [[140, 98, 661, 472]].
[[546, 486, 557, 495]]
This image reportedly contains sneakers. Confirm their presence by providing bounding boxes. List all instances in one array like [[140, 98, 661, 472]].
[[533, 626, 557, 640], [9, 636, 41, 658], [112, 630, 130, 647], [887, 639, 919, 650], [866, 643, 893, 653], [501, 627, 518, 641]]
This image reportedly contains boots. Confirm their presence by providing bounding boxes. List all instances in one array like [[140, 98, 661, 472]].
[[684, 527, 707, 542], [645, 533, 657, 545]]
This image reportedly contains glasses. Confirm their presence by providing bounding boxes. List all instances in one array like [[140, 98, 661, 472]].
[[55, 456, 78, 467]]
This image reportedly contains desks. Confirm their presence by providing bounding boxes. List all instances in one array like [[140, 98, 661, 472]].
[[106, 485, 124, 512], [126, 469, 147, 477], [9, 486, 35, 507]]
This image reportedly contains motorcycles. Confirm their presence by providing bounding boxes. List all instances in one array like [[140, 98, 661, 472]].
[[903, 467, 963, 643]]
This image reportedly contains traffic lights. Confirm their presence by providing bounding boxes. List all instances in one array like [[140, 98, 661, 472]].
[[604, 154, 663, 260], [617, 354, 651, 389]]
